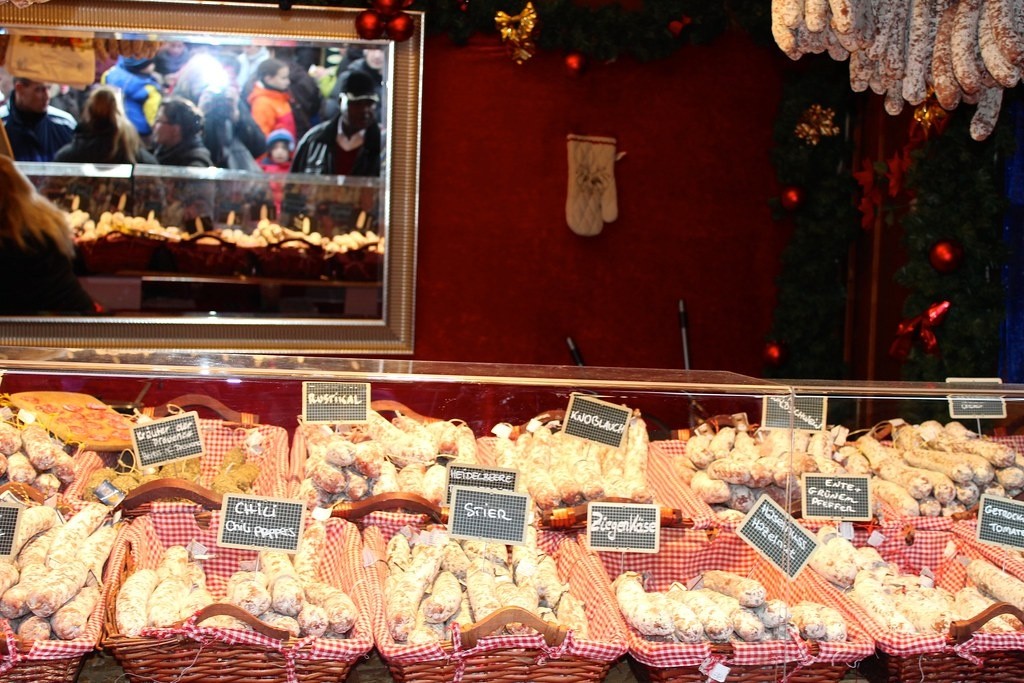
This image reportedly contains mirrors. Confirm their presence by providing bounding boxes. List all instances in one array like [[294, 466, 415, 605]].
[[0, 2, 426, 353]]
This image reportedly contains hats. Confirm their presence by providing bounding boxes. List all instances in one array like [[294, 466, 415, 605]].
[[267, 129, 294, 151], [337, 70, 380, 104], [118, 52, 155, 73]]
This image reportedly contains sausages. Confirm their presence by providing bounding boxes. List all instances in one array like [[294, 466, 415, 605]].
[[770, 0, 1024, 141], [1, 415, 1024, 639]]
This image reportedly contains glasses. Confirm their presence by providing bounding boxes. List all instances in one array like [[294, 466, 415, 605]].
[[153, 116, 179, 127]]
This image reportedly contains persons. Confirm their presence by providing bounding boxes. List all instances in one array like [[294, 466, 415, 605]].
[[0, 34, 388, 315]]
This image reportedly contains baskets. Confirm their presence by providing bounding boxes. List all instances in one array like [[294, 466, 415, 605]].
[[58, 212, 385, 284], [0, 390, 1024, 683]]
